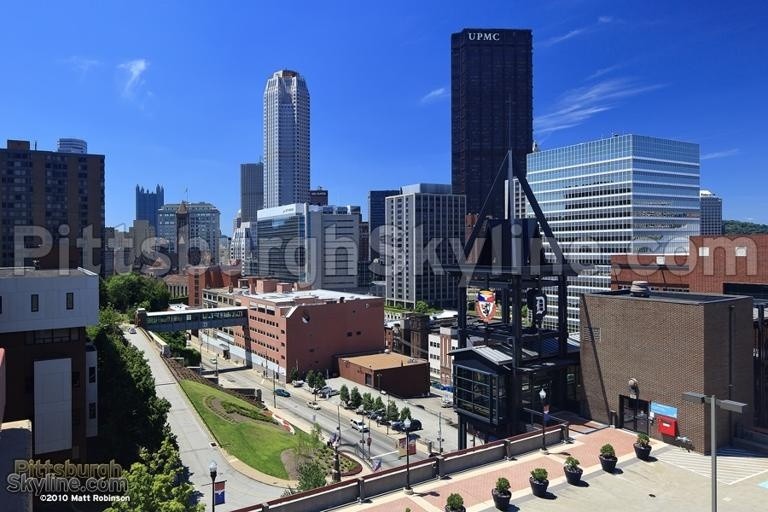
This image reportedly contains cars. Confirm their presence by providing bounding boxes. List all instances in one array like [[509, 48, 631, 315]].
[[210, 358, 216, 364], [430, 379, 453, 393], [127, 326, 136, 334], [275, 388, 290, 397], [340, 398, 422, 433], [306, 400, 320, 410]]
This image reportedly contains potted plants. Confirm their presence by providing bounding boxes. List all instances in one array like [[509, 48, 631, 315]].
[[442, 432, 652, 512]]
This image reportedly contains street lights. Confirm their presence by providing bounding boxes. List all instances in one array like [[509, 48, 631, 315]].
[[682, 388, 751, 512], [402, 415, 413, 490], [200, 459, 227, 511], [200, 342, 204, 364], [366, 408, 383, 462], [380, 389, 391, 435], [538, 388, 548, 450]]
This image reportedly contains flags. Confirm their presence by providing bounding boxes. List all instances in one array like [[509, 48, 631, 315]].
[[543, 404, 550, 423], [214, 480, 225, 506], [408, 432, 417, 455], [397, 437, 408, 459]]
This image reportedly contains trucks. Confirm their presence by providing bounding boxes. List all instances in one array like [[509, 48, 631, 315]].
[[440, 397, 453, 408]]
